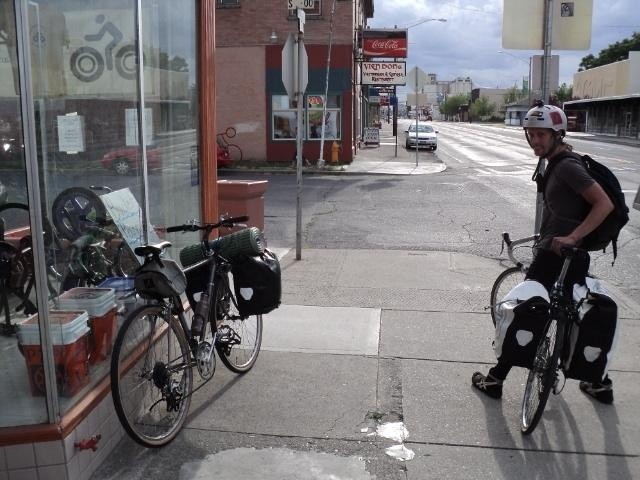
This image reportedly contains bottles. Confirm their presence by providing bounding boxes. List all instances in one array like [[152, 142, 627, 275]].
[[191, 294, 211, 339]]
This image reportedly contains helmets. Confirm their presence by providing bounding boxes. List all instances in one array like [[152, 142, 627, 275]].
[[522, 106, 567, 136]]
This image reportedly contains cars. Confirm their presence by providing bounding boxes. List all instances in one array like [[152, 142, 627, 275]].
[[102, 129, 233, 176], [405, 124, 439, 151]]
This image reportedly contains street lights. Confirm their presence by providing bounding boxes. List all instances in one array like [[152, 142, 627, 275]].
[[497, 49, 520, 61], [405, 18, 448, 30]]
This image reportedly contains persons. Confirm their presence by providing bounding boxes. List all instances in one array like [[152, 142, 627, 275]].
[[471, 104, 614, 405]]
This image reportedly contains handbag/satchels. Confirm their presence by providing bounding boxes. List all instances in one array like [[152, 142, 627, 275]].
[[561, 278, 617, 382], [232, 249, 282, 318], [491, 280, 551, 369], [185, 249, 229, 318]]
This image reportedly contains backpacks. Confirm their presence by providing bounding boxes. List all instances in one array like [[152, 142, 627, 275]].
[[543, 151, 629, 251]]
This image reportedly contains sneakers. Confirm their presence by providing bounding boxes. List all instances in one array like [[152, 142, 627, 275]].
[[471, 371, 502, 397], [579, 380, 614, 404]]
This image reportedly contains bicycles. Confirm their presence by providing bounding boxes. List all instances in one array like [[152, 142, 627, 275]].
[[110, 215, 261, 448], [0, 185, 141, 334], [490, 233, 589, 436]]
